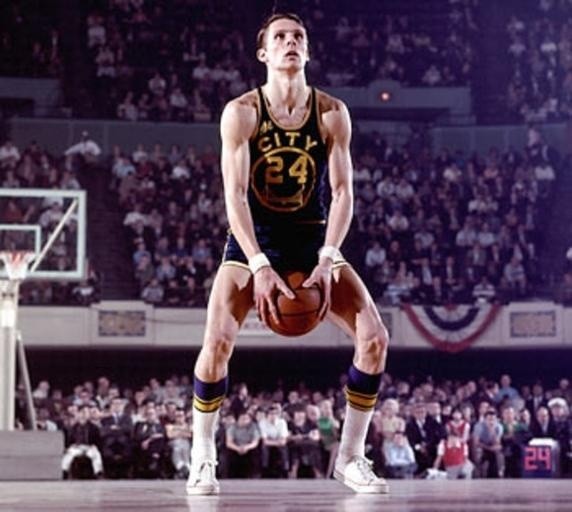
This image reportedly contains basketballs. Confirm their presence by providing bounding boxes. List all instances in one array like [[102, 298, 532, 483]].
[[264, 269, 321, 336]]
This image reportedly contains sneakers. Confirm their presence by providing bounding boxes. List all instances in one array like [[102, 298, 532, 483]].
[[186, 452, 219, 495], [333, 455, 389, 495]]
